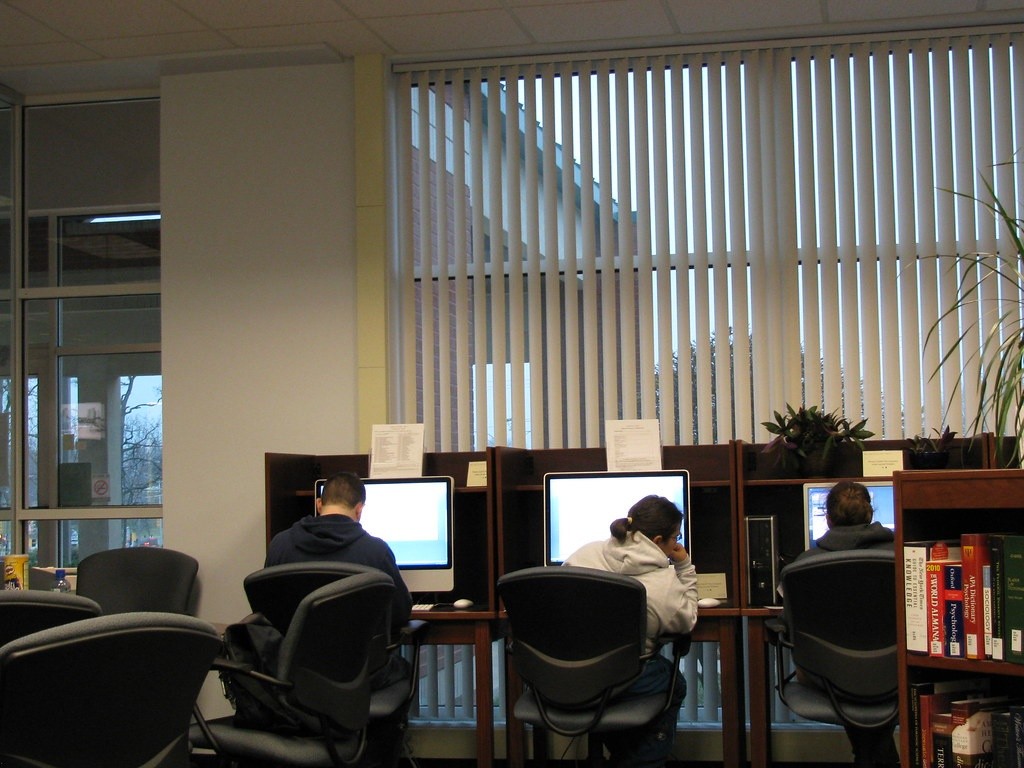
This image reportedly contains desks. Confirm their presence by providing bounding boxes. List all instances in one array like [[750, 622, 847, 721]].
[[263, 431, 1021, 768]]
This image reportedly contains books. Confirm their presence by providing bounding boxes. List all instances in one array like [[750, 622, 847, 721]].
[[909, 679, 1024, 768], [904, 533, 1024, 664]]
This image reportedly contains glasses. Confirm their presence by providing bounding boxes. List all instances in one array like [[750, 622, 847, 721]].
[[665, 532, 681, 541]]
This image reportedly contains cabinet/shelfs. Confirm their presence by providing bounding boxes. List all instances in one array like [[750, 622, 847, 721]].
[[893, 469, 1024, 768]]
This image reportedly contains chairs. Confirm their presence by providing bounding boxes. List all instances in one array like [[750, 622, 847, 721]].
[[497, 565, 692, 768], [0, 548, 426, 768], [767, 549, 897, 729]]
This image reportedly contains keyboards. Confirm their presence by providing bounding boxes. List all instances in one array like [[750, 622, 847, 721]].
[[411, 604, 435, 612]]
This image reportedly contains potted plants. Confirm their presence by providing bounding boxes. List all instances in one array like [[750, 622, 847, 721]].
[[900, 425, 964, 469], [759, 403, 876, 479]]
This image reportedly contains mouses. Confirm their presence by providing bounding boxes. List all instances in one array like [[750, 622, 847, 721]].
[[454, 598, 474, 608], [697, 597, 721, 608]]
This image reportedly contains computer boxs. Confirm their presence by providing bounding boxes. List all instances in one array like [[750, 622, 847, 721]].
[[745, 514, 780, 607]]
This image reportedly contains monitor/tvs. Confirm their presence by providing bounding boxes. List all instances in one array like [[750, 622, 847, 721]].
[[803, 480, 895, 552], [314, 476, 454, 592], [543, 468, 691, 568]]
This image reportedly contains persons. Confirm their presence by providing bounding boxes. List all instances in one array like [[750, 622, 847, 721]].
[[774, 480, 901, 768], [523, 494, 700, 768], [262, 472, 414, 691]]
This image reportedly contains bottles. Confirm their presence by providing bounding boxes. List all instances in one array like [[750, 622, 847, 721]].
[[49, 569, 72, 593]]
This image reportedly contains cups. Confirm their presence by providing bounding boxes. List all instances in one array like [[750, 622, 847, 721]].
[[0, 554, 30, 590]]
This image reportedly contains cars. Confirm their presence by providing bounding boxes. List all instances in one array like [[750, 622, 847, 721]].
[[71, 529, 78, 546]]
